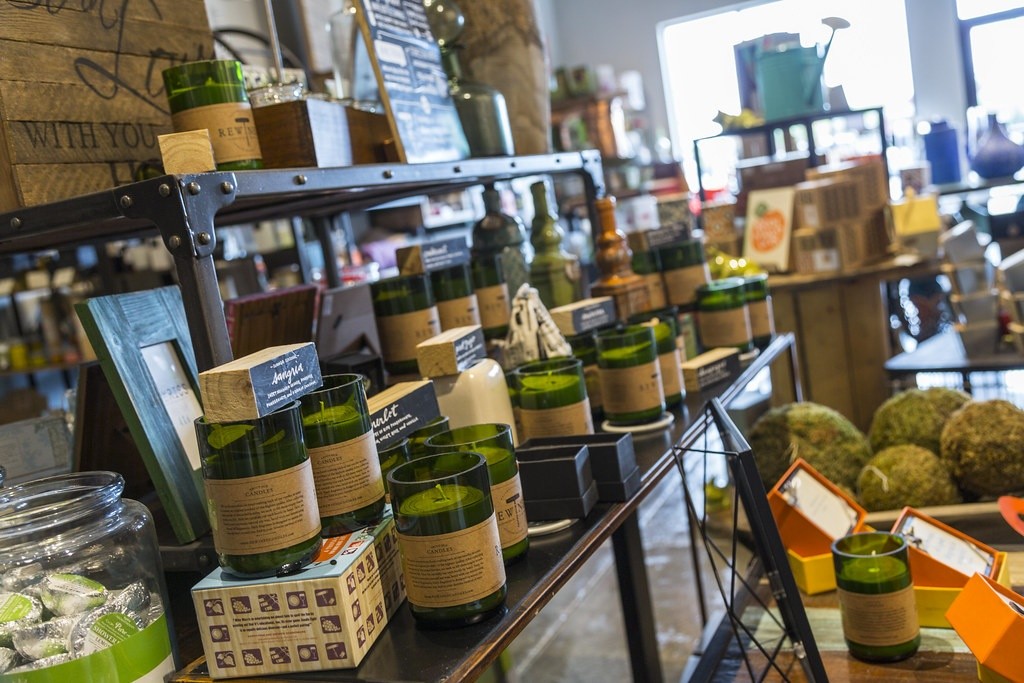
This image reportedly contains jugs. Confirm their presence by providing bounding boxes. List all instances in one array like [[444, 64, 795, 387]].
[[754, 17, 850, 122]]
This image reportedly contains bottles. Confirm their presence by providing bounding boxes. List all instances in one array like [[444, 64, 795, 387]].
[[922, 121, 962, 185], [324, 0, 388, 115], [0, 470, 176, 683], [553, 65, 674, 165], [969, 114, 1024, 179], [439, 44, 516, 157]]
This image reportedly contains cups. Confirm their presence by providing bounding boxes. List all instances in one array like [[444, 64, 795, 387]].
[[939, 220, 1024, 360], [245, 78, 303, 109]]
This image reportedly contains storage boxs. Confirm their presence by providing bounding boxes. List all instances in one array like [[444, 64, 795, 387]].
[[944, 571, 1024, 683], [889, 505, 998, 587], [190, 504, 406, 681], [766, 456, 868, 556]]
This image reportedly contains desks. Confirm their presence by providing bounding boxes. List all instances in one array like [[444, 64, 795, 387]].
[[882, 320, 1024, 397]]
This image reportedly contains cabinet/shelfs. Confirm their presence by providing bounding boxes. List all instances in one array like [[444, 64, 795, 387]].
[[0, 0, 1024, 683]]
